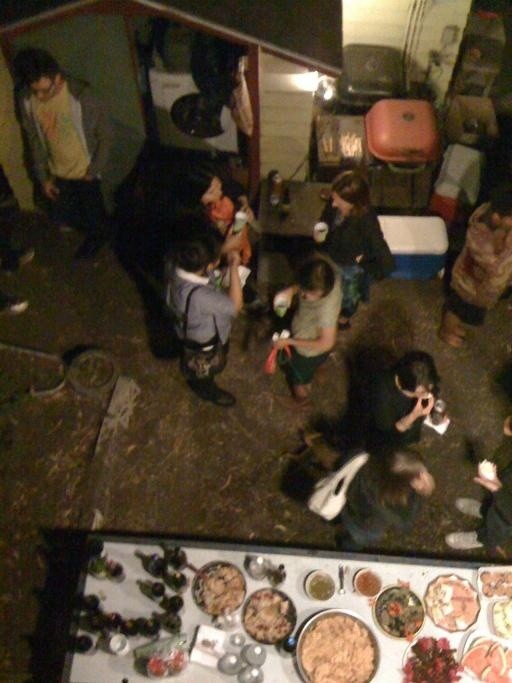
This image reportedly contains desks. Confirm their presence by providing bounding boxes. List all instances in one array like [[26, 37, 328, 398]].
[[461, 60, 500, 99], [258, 179, 335, 271], [308, 115, 415, 208]]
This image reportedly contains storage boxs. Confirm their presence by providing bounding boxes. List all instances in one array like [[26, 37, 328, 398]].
[[377, 216, 450, 280], [428, 143, 485, 220]]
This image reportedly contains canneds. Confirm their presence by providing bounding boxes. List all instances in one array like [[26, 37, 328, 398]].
[[433, 399, 448, 426]]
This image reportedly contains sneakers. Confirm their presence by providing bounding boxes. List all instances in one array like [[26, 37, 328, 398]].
[[455, 497, 484, 518], [445, 529, 484, 549]]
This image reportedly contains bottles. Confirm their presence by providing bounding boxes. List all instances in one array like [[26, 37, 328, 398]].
[[214, 632, 297, 683], [269, 173, 293, 218], [273, 562, 286, 584], [248, 553, 270, 580], [213, 268, 222, 292], [218, 614, 237, 629], [70, 539, 193, 657]]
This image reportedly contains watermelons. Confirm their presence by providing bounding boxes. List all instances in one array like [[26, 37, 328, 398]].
[[461, 639, 512, 683]]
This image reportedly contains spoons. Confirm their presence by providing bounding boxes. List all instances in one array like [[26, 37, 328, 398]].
[[201, 639, 219, 658], [338, 564, 347, 595]]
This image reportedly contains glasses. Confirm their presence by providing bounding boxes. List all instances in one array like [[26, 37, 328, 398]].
[[33, 81, 54, 94]]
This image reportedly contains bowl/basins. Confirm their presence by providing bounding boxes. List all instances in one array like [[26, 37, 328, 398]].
[[297, 607, 381, 683]]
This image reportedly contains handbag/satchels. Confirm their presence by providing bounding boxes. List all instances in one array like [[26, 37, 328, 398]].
[[180, 333, 227, 380], [264, 345, 292, 375], [307, 451, 369, 520]]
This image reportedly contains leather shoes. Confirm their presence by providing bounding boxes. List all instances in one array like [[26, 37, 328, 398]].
[[212, 388, 236, 405], [74, 242, 97, 258]]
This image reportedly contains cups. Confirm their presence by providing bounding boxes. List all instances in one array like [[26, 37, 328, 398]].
[[313, 221, 329, 242], [276, 302, 287, 318], [234, 211, 247, 232], [144, 649, 191, 681]]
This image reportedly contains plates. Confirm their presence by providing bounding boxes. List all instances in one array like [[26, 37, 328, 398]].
[[371, 563, 511, 683], [193, 559, 248, 618], [354, 566, 384, 598], [305, 569, 337, 603], [239, 587, 297, 645]]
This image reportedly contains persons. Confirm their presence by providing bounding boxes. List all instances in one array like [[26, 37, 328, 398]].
[[367, 351, 439, 447], [11, 47, 117, 260], [338, 449, 436, 552], [183, 164, 270, 316], [438, 189, 512, 349], [166, 225, 243, 406], [273, 252, 343, 404], [0, 247, 36, 315], [319, 170, 381, 330], [445, 459, 512, 550]]
